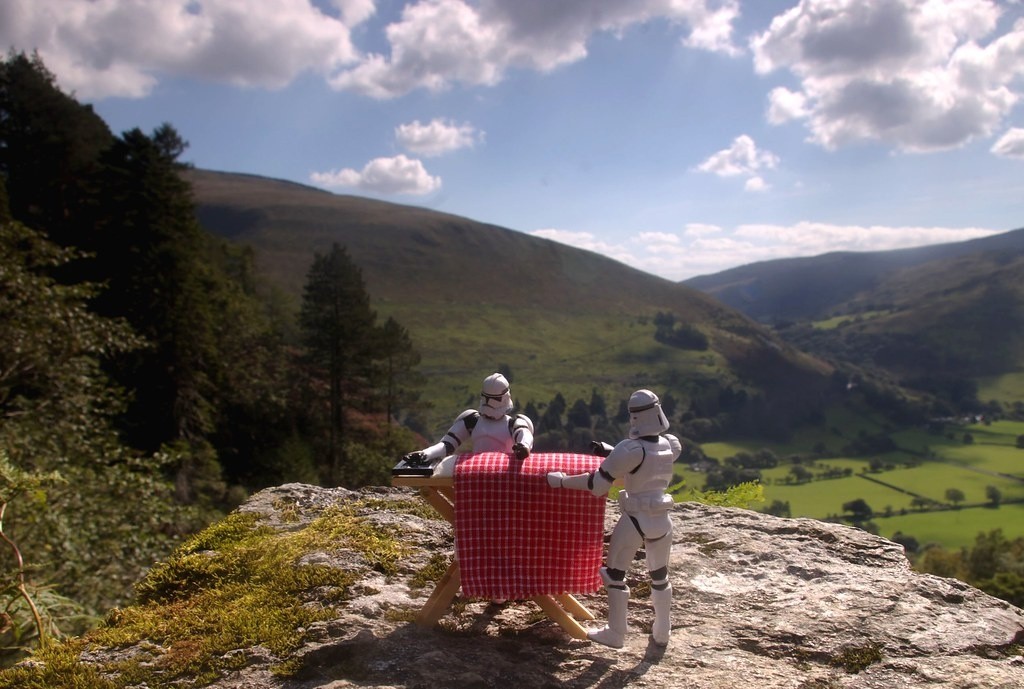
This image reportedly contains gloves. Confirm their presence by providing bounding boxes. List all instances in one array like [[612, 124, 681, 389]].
[[547, 472, 563, 488], [403, 451, 428, 466], [512, 442, 530, 459], [590, 440, 611, 457]]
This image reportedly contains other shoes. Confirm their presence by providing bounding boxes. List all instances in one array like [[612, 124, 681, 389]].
[[587, 625, 624, 648], [652, 622, 671, 646]]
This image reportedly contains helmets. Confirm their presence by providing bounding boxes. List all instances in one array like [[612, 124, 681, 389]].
[[628, 389, 670, 439], [478, 373, 513, 419]]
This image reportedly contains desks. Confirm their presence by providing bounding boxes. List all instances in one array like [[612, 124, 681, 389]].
[[389, 459, 624, 639]]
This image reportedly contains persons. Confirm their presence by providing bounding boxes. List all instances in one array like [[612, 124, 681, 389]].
[[547, 389, 681, 649], [403, 373, 534, 604]]
